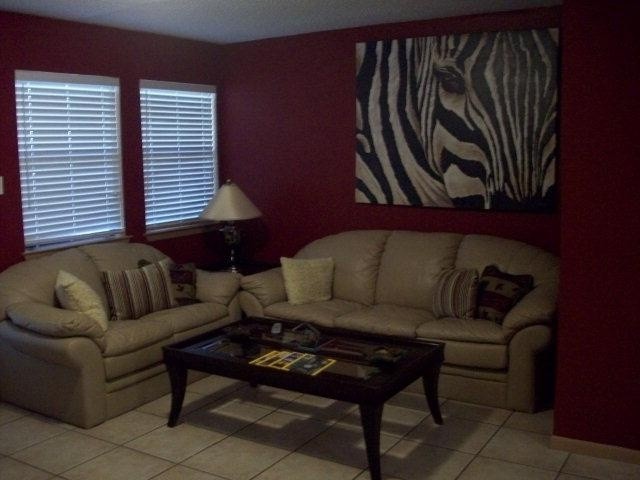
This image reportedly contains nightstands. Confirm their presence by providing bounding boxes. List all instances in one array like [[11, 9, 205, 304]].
[[209, 261, 280, 276]]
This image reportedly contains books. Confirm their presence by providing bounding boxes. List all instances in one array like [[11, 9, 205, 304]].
[[249, 349, 337, 378]]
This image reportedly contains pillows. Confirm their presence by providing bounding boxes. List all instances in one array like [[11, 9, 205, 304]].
[[432, 266, 480, 319], [280, 257, 335, 304], [139, 258, 201, 306], [55, 269, 108, 332], [476, 264, 533, 326], [103, 259, 179, 321]]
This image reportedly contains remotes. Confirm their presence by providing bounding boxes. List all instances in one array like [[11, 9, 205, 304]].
[[271, 322, 282, 334]]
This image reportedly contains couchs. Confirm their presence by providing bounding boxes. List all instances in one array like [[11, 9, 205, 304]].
[[239, 229, 561, 414], [0, 242, 243, 430]]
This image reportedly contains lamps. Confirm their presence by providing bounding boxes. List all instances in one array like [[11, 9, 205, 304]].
[[200, 178, 264, 272]]
